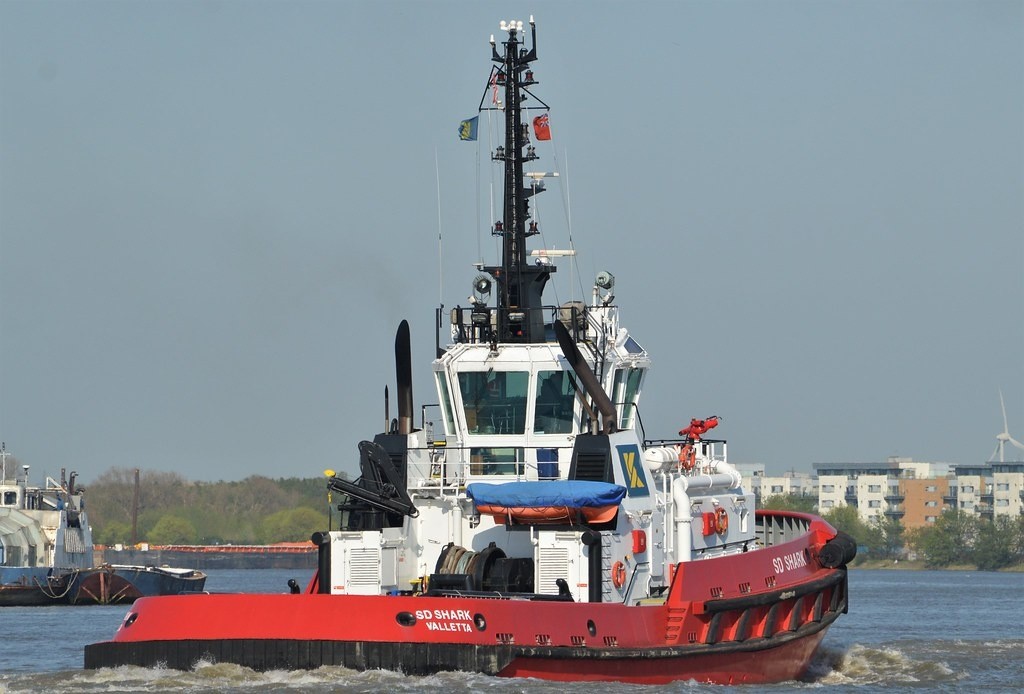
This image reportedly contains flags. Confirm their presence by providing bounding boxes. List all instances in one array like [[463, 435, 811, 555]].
[[533, 113, 551, 141], [493, 73, 498, 104], [458, 116, 478, 141]]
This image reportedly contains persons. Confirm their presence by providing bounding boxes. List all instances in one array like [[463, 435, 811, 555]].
[[476, 398, 489, 433], [287, 579, 300, 594]]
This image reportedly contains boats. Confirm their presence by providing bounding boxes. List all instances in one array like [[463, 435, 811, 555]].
[[79, 14, 857, 685], [0, 438, 208, 605]]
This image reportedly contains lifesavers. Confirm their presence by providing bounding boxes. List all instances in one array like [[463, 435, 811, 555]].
[[681, 445, 696, 470], [713, 506, 730, 533], [612, 561, 627, 588]]
